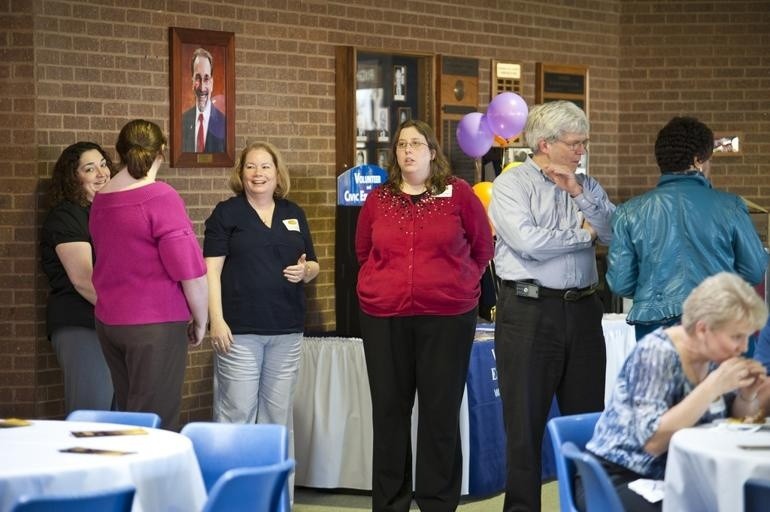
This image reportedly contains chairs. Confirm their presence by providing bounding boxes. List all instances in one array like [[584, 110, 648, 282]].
[[561, 438, 626, 512], [201, 459, 300, 511], [64, 407, 160, 427], [8, 485, 139, 511], [546, 410, 606, 512], [177, 419, 290, 510]]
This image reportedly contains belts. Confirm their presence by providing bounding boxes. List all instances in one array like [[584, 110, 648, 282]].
[[504, 280, 596, 302]]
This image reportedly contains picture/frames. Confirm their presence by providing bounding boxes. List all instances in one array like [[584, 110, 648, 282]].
[[168, 25, 237, 167], [356, 59, 413, 171]]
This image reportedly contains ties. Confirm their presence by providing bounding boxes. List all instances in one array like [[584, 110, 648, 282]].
[[197, 113, 204, 153]]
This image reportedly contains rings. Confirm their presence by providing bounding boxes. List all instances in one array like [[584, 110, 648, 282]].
[[214, 342, 218, 344]]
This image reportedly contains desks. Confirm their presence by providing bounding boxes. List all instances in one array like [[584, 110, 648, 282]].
[[292, 313, 636, 500]]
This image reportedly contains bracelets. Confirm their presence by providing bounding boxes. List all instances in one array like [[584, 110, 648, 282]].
[[739, 392, 759, 403]]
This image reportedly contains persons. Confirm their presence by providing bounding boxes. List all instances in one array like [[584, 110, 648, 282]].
[[356, 121, 493, 510], [358, 62, 409, 168], [39, 142, 115, 420], [605, 118, 769, 351], [200, 139, 320, 510], [90, 118, 208, 426], [492, 96, 629, 511], [593, 270, 769, 512], [180, 49, 228, 153]]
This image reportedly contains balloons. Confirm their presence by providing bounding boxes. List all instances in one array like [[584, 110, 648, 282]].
[[469, 178, 499, 235], [489, 90, 527, 143], [506, 161, 522, 172], [456, 113, 490, 157]]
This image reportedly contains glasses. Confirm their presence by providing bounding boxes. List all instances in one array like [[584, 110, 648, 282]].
[[394, 140, 429, 148], [558, 138, 589, 150]]
